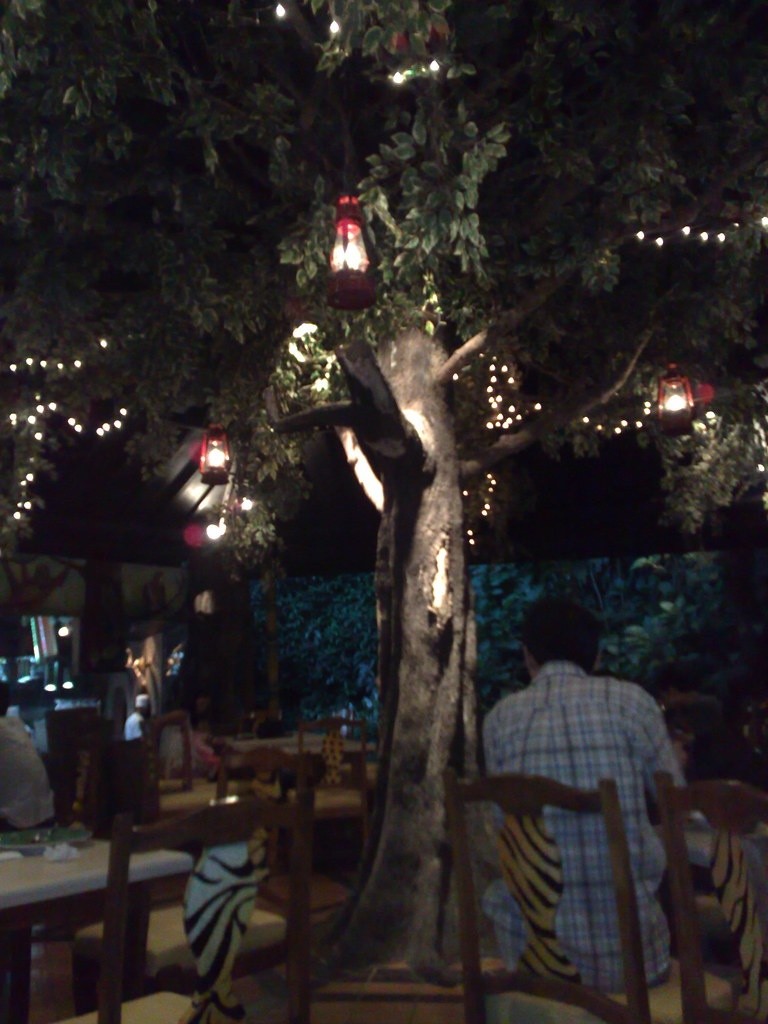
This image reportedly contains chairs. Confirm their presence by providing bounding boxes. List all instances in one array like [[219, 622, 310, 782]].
[[0, 707, 768, 1024]]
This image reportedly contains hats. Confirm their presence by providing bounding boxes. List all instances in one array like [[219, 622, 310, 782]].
[[134, 694, 149, 708]]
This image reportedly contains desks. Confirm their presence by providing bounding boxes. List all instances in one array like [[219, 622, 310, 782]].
[[155, 777, 370, 824], [216, 731, 376, 769], [0, 836, 193, 1024]]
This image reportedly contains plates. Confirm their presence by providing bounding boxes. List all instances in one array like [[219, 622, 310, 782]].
[[0, 827, 95, 857]]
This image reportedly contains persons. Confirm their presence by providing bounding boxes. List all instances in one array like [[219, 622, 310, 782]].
[[91, 648, 148, 839], [483, 595, 691, 993], [0, 681, 57, 834], [644, 690, 768, 983], [157, 687, 216, 782]]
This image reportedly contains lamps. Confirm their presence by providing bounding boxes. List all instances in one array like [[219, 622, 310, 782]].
[[657, 362, 697, 438], [200, 423, 229, 487], [327, 187, 377, 310]]
